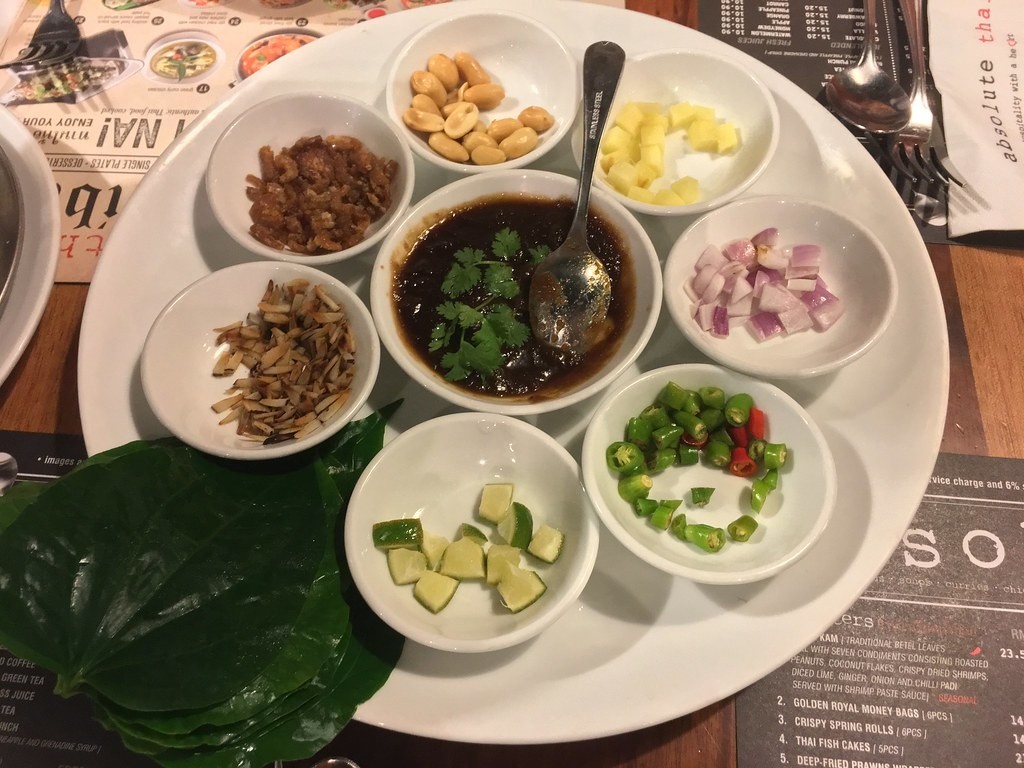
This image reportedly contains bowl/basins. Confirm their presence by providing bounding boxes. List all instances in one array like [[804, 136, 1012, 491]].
[[370, 168, 662, 414], [140, 31, 226, 86]]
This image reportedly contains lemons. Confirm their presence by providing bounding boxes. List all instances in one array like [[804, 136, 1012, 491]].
[[373, 484, 564, 613]]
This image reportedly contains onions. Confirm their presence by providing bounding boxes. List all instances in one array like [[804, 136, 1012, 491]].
[[683, 228, 844, 343]]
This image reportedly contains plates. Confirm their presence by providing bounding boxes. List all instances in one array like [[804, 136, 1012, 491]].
[[345, 412, 601, 654], [0, 105, 62, 389], [582, 363, 839, 585], [78, 0, 952, 747], [204, 91, 415, 265], [0, 56, 144, 105], [387, 11, 580, 174], [568, 51, 780, 218], [141, 261, 381, 459], [237, 33, 320, 81], [663, 196, 898, 379]]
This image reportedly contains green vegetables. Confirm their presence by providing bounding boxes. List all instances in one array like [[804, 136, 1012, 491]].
[[427, 227, 555, 385]]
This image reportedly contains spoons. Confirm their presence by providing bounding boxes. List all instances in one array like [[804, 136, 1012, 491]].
[[826, 0, 912, 133], [528, 41, 626, 353]]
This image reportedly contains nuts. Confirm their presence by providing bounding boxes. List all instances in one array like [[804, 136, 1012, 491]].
[[403, 53, 554, 165]]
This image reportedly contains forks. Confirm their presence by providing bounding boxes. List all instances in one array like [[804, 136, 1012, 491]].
[[888, 0, 969, 187], [0, 0, 82, 69]]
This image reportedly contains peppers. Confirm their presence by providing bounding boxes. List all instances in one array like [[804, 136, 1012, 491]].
[[607, 381, 787, 551]]
